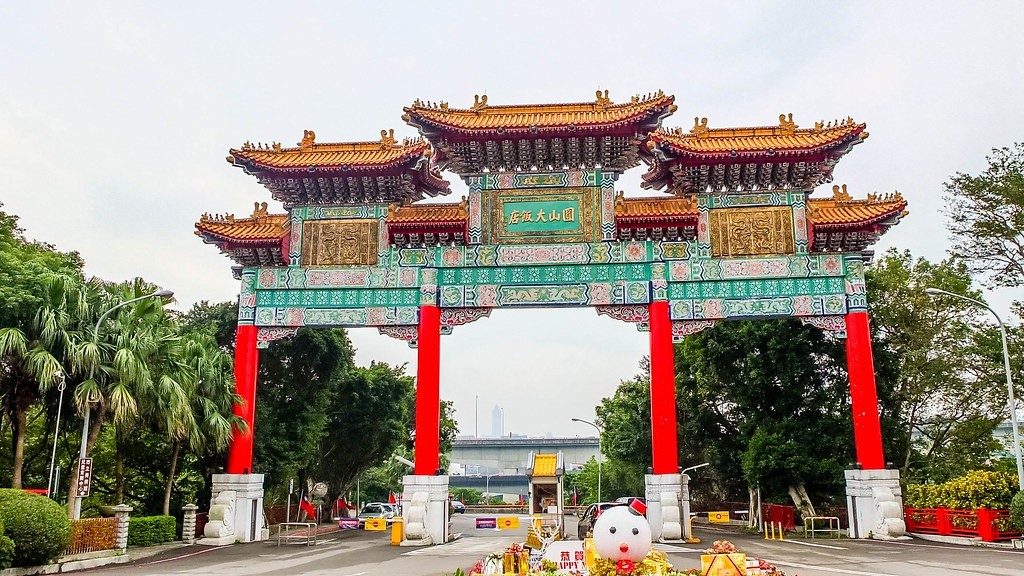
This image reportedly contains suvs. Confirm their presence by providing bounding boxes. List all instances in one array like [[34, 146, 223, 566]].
[[577, 501, 627, 540]]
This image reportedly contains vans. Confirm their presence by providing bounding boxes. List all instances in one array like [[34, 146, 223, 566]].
[[613, 497, 645, 505]]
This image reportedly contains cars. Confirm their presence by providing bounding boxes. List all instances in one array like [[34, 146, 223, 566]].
[[357, 502, 400, 529], [449, 500, 466, 514]]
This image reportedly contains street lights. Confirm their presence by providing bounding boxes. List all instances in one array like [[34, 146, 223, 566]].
[[475, 395, 478, 444], [570, 417, 602, 502], [486, 473, 499, 505], [500, 407, 505, 440], [924, 287, 1024, 491], [73, 289, 175, 521], [46, 369, 66, 499]]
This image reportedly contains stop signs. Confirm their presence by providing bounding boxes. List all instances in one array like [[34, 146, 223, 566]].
[[373, 522, 378, 527], [505, 520, 511, 526]]
